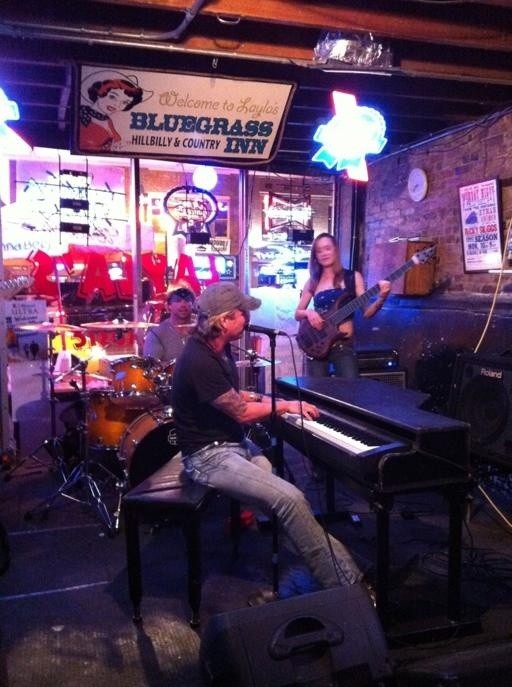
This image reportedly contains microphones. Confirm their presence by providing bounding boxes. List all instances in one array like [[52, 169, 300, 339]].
[[245, 325, 288, 336]]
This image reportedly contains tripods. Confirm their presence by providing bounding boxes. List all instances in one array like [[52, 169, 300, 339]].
[[35, 397, 121, 536], [4, 348, 73, 482]]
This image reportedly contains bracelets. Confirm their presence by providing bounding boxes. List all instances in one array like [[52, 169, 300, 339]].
[[257, 394, 263, 402], [249, 392, 254, 399]]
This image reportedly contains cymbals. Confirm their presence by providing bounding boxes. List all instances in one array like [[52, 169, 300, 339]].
[[233, 358, 280, 368], [175, 323, 196, 329], [80, 322, 162, 331], [16, 324, 84, 332]]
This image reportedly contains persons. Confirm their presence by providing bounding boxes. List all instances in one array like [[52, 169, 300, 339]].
[[172, 283, 380, 608], [295, 233, 391, 378], [143, 283, 196, 364], [79, 70, 153, 150]]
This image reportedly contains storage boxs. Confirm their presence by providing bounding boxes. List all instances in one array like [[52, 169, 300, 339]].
[[370, 239, 438, 295]]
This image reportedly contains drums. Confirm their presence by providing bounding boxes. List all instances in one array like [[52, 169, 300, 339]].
[[116, 403, 181, 492], [86, 388, 147, 450], [109, 356, 163, 409], [154, 357, 177, 402]]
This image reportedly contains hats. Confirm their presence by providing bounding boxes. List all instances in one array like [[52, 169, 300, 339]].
[[195, 281, 261, 315]]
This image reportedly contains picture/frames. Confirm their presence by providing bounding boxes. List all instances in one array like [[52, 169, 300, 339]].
[[458, 178, 504, 274]]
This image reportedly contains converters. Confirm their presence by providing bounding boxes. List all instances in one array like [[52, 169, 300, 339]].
[[350, 513, 361, 524]]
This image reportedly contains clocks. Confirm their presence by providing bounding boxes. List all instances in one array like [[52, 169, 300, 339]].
[[407, 168, 428, 202]]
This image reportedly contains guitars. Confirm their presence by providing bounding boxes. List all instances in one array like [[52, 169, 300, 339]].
[[297, 248, 435, 361]]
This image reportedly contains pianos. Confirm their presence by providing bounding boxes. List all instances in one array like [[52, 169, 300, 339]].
[[260, 376, 483, 648]]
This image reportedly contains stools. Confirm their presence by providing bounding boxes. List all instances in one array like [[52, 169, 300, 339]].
[[122, 450, 241, 628]]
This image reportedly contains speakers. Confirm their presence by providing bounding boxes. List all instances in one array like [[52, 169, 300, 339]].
[[444, 351, 512, 470], [199, 583, 394, 687]]
